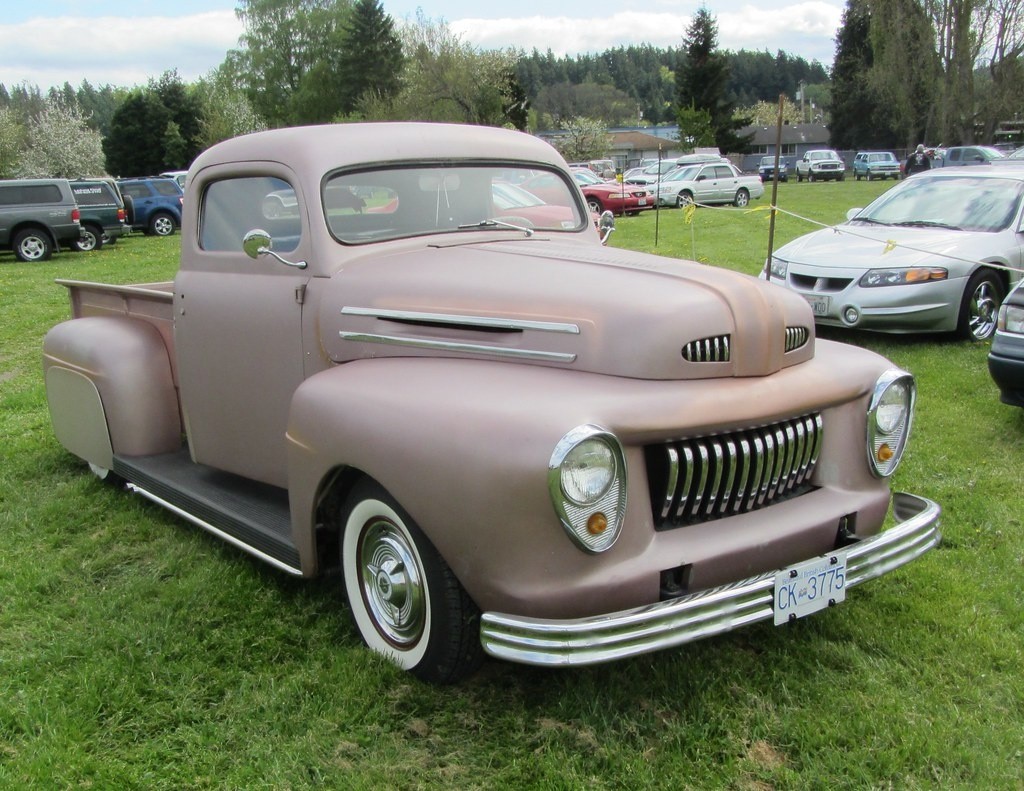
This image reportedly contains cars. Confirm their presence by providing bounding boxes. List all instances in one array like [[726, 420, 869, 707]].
[[994, 142, 1015, 157], [1008, 143, 1024, 161], [984, 277, 1024, 409], [568, 152, 764, 210], [366, 176, 615, 248], [756, 163, 1024, 341], [263, 188, 299, 218], [516, 167, 655, 216], [756, 155, 790, 182]]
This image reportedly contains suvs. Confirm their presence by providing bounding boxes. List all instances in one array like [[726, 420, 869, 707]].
[[0, 178, 86, 263], [67, 179, 131, 252], [66, 178, 136, 238], [115, 176, 184, 236]]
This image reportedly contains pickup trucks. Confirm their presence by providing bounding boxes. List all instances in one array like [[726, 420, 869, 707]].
[[898, 143, 1006, 175], [39, 119, 944, 689], [796, 150, 846, 182]]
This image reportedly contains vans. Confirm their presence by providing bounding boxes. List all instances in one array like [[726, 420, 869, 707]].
[[852, 151, 900, 181], [159, 170, 189, 190]]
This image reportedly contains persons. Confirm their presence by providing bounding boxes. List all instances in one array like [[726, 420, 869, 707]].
[[904, 144, 931, 177]]
